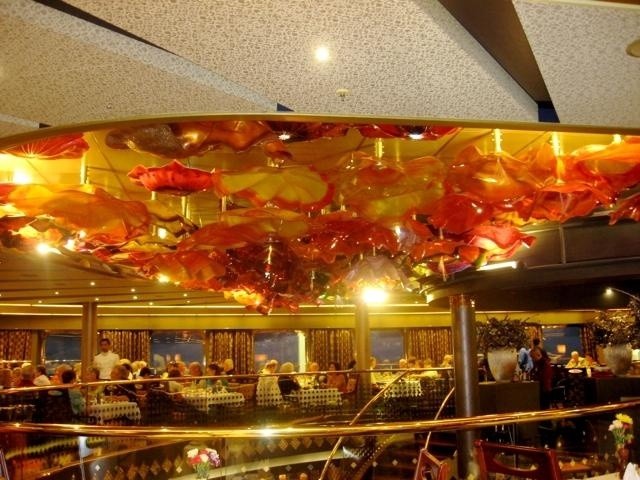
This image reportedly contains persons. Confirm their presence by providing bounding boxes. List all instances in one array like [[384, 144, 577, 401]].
[[528, 347, 546, 387], [399, 353, 486, 385], [532, 339, 552, 364], [0, 356, 359, 431], [92, 337, 120, 381], [564, 351, 592, 368], [369, 357, 380, 386]]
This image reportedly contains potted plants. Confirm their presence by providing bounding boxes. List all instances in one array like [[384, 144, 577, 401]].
[[471, 313, 531, 382], [588, 297, 640, 374]]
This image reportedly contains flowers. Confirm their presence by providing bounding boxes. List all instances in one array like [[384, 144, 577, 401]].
[[185, 446, 220, 479], [608, 413, 634, 451]]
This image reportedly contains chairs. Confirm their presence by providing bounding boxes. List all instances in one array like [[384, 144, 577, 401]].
[[40, 387, 72, 423], [413, 447, 451, 480], [84, 372, 456, 426], [473, 438, 563, 479]]
